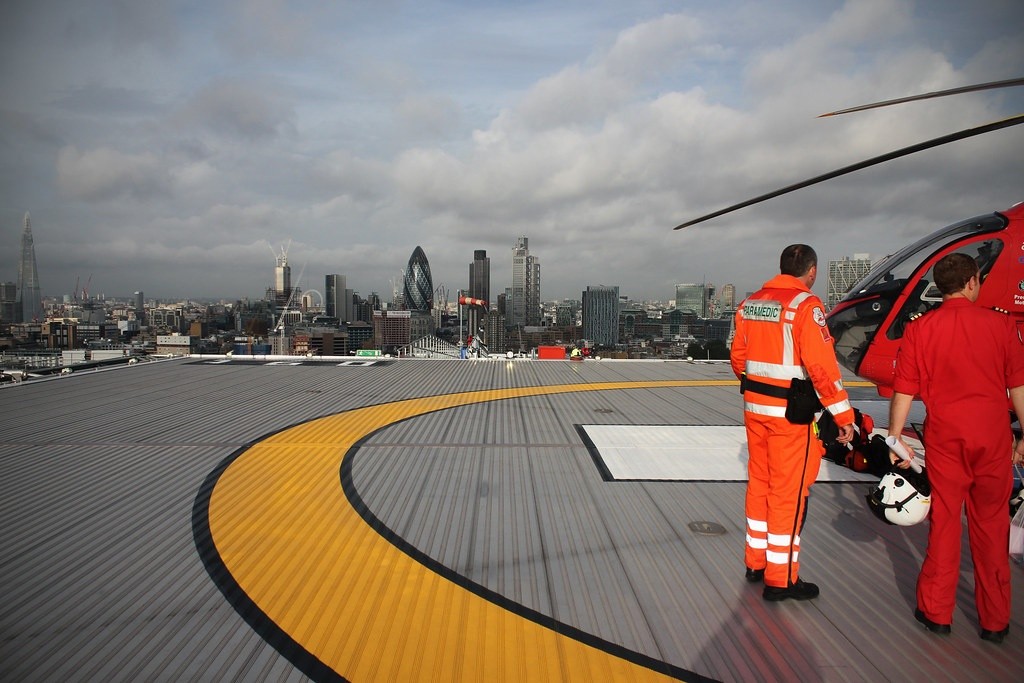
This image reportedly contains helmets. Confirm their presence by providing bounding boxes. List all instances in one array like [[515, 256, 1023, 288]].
[[1010, 478, 1024, 518], [865, 459, 932, 526]]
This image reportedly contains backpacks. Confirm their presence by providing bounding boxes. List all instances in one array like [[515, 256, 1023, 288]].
[[816, 407, 892, 478]]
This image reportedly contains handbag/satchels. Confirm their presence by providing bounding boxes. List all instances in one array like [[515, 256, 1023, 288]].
[[1009, 500, 1024, 569]]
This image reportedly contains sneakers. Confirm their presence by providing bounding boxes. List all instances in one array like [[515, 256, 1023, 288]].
[[745, 567, 764, 582], [914, 608, 951, 634], [980, 623, 1010, 644], [763, 576, 820, 602]]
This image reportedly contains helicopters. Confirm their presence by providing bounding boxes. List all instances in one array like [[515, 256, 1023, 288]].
[[669, 72, 1024, 484]]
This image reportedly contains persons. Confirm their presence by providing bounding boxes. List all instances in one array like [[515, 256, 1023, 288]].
[[888, 252, 1024, 645], [728, 242, 858, 600], [571, 346, 581, 356]]
